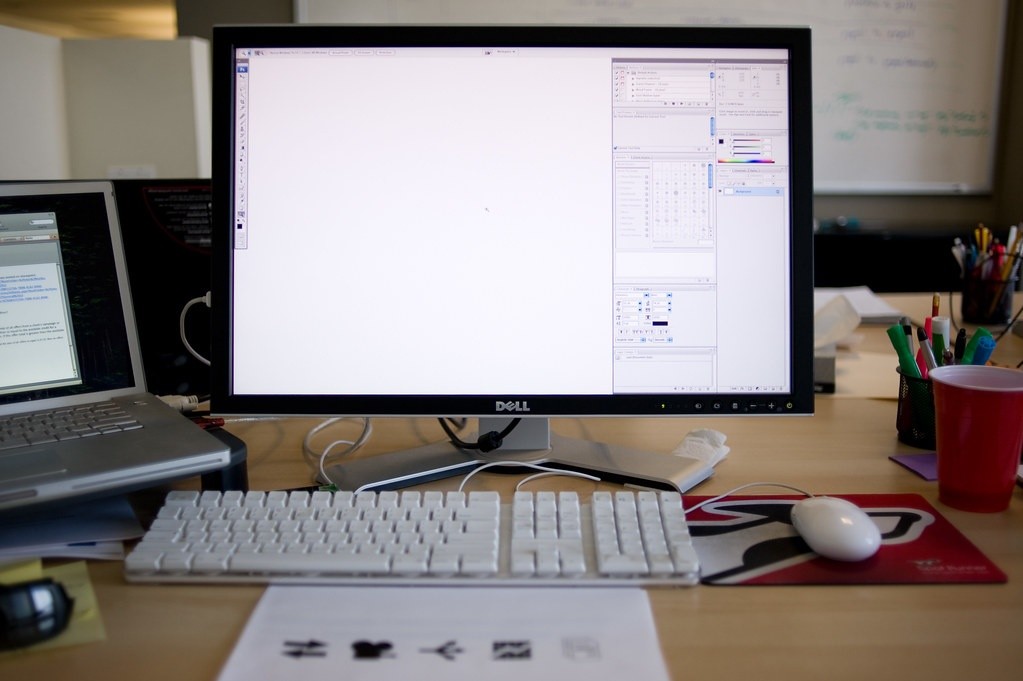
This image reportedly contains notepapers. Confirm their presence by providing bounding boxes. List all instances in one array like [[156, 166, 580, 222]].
[[889, 454, 938, 481]]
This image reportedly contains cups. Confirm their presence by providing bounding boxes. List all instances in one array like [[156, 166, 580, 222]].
[[928, 364, 1022, 512]]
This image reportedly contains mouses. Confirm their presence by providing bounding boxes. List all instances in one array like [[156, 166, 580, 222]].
[[791, 496, 879, 560]]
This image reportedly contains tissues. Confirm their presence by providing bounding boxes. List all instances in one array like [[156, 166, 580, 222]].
[[811, 289, 865, 398]]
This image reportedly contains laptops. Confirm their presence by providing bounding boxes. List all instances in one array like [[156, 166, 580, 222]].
[[0, 181, 230, 511]]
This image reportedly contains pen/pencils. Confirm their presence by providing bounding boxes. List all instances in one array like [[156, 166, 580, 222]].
[[953, 222, 1023, 324], [887, 292, 994, 450]]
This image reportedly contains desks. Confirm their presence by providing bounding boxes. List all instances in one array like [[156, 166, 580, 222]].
[[0, 290, 1022, 679]]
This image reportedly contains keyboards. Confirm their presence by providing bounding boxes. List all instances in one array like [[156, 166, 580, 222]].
[[122, 488, 703, 587]]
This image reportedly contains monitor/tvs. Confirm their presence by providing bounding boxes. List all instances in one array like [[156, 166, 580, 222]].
[[210, 24, 816, 417]]
